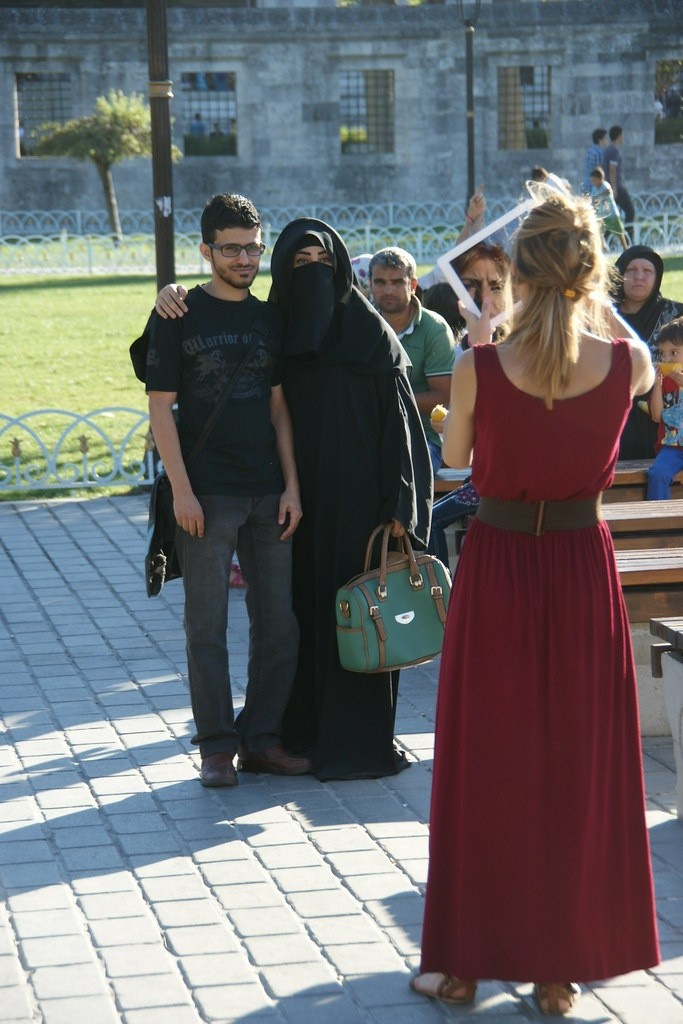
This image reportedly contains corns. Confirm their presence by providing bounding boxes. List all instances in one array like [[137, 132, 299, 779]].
[[658, 362, 682, 375], [431, 407, 447, 422], [637, 400, 648, 414]]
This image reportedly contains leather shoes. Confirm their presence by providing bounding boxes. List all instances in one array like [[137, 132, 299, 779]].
[[200, 753, 240, 786], [237, 748, 310, 774]]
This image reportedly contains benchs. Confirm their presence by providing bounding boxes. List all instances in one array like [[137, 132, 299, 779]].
[[432, 458, 683, 818]]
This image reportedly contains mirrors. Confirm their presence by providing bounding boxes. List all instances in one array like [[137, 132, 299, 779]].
[[438, 198, 547, 332]]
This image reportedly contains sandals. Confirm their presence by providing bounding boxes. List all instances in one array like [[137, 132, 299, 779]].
[[410, 970, 477, 1005], [535, 980, 580, 1015]]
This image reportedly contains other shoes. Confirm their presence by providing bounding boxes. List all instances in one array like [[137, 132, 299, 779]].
[[229, 564, 249, 588]]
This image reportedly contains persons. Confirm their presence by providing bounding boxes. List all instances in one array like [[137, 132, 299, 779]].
[[411, 195, 661, 1016], [526, 120, 547, 149], [609, 244, 683, 502], [582, 125, 636, 253], [190, 112, 236, 138], [155, 218, 433, 780], [145, 195, 312, 787], [348, 246, 484, 575], [450, 236, 512, 320], [530, 166, 572, 196]]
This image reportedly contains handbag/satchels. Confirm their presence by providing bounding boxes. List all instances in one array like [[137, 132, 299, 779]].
[[145, 469, 181, 596], [334, 523, 455, 671]]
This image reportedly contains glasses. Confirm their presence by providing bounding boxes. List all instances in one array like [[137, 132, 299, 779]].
[[206, 241, 266, 257]]
